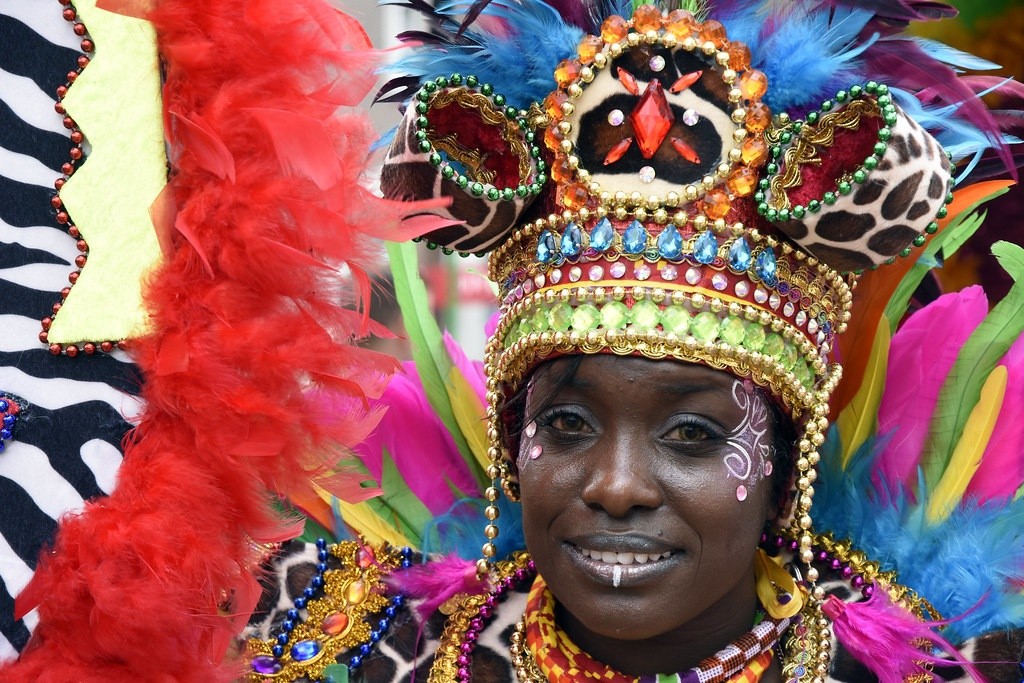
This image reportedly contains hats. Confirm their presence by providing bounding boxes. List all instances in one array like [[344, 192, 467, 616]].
[[351, 0, 1024, 421]]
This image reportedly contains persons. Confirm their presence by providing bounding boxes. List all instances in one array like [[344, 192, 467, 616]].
[[244, 211, 1024, 683]]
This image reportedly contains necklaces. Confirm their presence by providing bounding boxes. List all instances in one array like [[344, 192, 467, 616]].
[[431, 530, 935, 682]]
